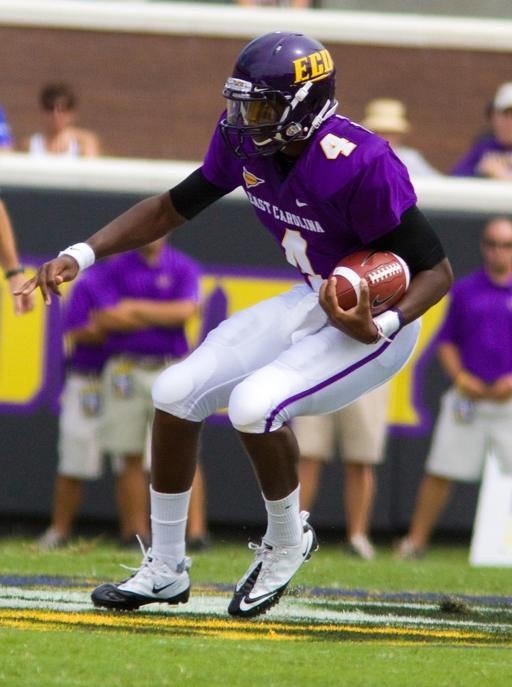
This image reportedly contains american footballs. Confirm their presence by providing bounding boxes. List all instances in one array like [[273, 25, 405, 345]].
[[329, 251, 411, 315]]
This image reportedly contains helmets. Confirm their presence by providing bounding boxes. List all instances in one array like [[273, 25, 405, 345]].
[[222, 34, 335, 161]]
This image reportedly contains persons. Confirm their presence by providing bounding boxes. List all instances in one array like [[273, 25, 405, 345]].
[[357, 96, 446, 178], [0, 199, 38, 320], [17, 80, 100, 158], [451, 80, 511, 180], [291, 215, 512, 565], [13, 29, 459, 618], [32, 228, 227, 552]]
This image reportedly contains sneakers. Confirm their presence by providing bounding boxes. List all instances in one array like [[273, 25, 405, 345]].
[[90, 555, 192, 611], [229, 511, 319, 617]]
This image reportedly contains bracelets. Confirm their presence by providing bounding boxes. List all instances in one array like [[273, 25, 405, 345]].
[[3, 266, 25, 279]]
